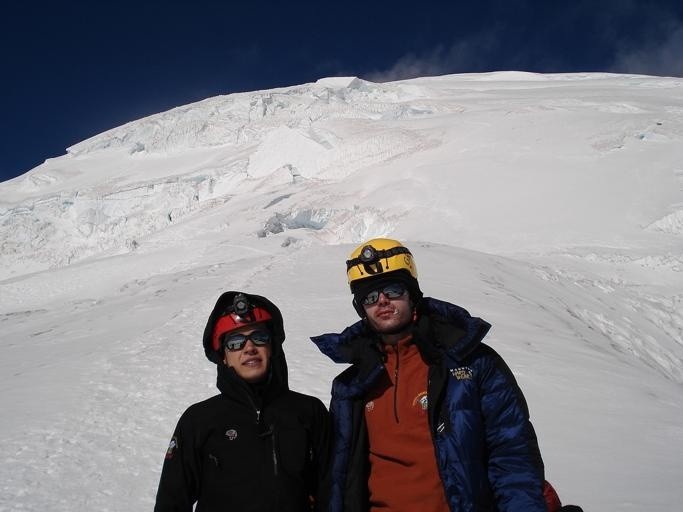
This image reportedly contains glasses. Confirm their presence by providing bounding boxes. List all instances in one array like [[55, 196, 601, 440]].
[[359, 284, 411, 305], [224, 328, 271, 352]]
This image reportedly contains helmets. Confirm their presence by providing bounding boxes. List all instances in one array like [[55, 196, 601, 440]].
[[346, 238, 419, 293], [212, 306, 274, 355]]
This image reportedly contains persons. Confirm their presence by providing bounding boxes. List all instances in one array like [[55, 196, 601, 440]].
[[152, 288, 339, 511], [308, 238, 580, 511]]
